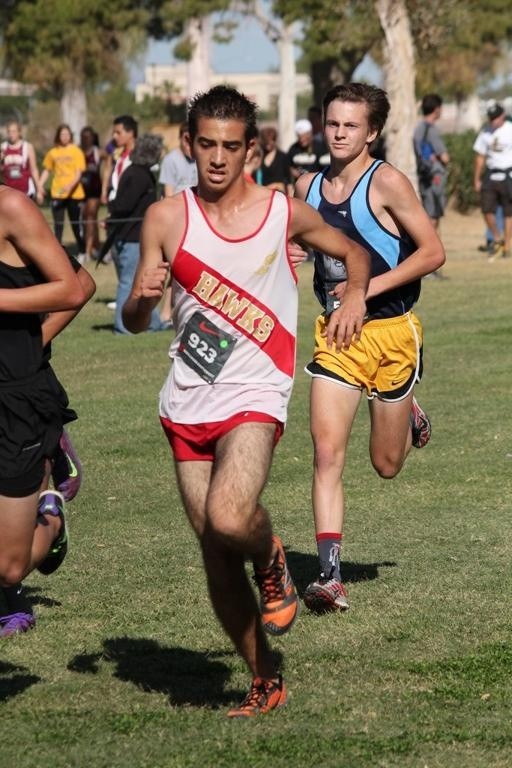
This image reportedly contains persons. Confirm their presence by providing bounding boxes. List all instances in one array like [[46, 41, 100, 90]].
[[0, 246, 96, 638], [479, 117, 512, 250], [0, 106, 386, 334], [288, 83, 446, 612], [122, 86, 372, 719], [0, 184, 84, 589], [413, 95, 450, 279], [472, 105, 512, 262]]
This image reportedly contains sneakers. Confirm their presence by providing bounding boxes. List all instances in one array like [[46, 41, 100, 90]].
[[302, 578, 350, 612], [1, 611, 34, 636], [35, 489, 70, 577], [410, 395, 432, 451], [50, 427, 83, 502], [254, 535, 299, 638], [227, 675, 288, 716]]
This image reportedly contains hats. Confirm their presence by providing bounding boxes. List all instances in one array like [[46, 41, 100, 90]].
[[486, 103, 504, 122], [294, 118, 314, 135]]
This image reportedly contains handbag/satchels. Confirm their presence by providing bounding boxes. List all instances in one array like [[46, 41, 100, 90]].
[[420, 141, 447, 197]]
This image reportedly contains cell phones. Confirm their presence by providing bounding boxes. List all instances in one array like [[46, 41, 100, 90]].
[[105, 139, 117, 154]]
